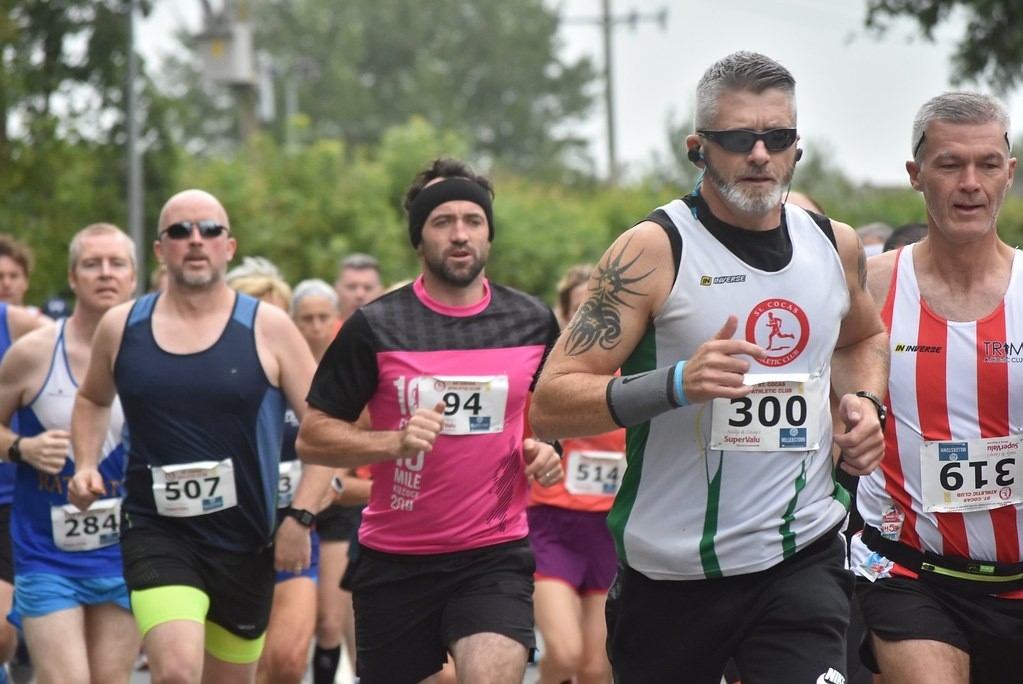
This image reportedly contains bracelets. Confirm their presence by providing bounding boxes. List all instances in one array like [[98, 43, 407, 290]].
[[675, 361, 691, 405]]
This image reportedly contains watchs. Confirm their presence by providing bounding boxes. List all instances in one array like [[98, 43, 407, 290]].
[[855, 390, 887, 434], [332, 476, 344, 494], [10, 436, 25, 463], [286, 508, 316, 527], [535, 438, 564, 460]]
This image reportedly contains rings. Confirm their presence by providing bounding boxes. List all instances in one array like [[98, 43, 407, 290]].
[[546, 473, 551, 479]]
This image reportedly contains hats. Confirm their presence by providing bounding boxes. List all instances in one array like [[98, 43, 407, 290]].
[[42, 298, 72, 319]]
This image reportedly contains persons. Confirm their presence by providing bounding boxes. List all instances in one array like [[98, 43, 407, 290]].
[[782, 91, 1023, 684], [295, 160, 566, 684], [528, 55, 891, 684], [0, 223, 167, 684], [71, 190, 338, 684], [527, 263, 629, 684], [226, 254, 418, 684]]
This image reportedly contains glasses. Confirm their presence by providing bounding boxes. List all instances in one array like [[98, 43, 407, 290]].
[[697, 128, 797, 153], [159, 220, 230, 240]]
